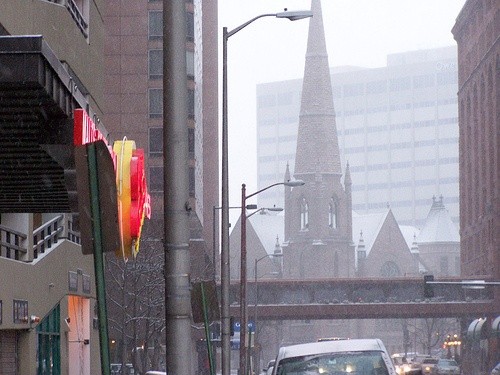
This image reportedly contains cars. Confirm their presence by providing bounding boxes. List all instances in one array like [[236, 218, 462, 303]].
[[262, 337, 462, 375]]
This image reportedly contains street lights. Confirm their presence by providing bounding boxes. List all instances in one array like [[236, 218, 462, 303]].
[[450, 334, 461, 361], [252, 253, 286, 375], [213, 205, 258, 312], [243, 207, 284, 375], [443, 333, 453, 359], [238, 179, 305, 375], [221, 10, 316, 375]]
